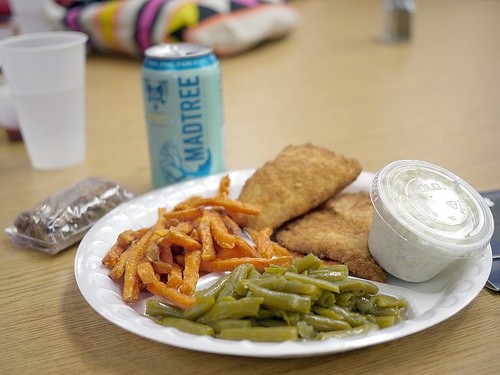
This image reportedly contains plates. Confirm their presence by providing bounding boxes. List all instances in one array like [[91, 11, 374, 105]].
[[74, 170, 492, 358]]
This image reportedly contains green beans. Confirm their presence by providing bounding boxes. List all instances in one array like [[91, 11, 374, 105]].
[[147, 253, 409, 344]]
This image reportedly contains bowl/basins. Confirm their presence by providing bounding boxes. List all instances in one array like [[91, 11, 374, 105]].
[[367, 160, 495, 281]]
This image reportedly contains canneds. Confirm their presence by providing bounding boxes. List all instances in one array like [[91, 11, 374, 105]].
[[139, 42, 228, 190]]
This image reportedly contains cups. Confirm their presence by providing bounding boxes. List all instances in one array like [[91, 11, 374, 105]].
[[0, 32, 88, 171], [1, 97, 22, 143]]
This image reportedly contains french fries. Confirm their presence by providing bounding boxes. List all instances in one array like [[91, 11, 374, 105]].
[[100, 176, 294, 310]]
